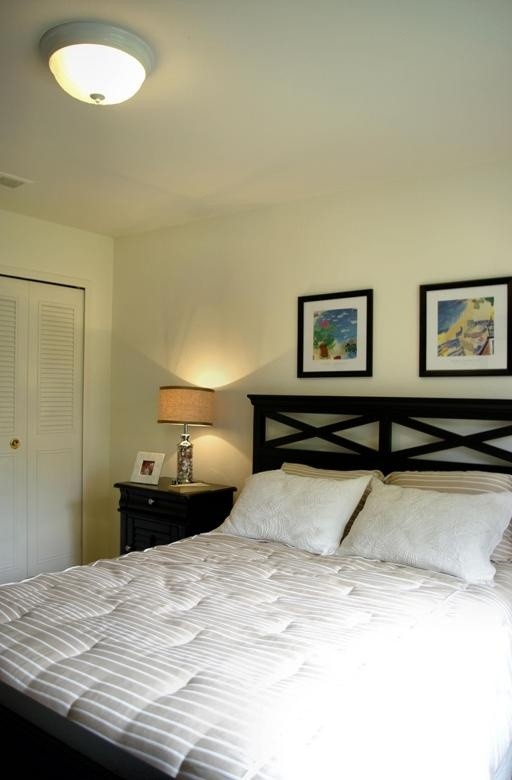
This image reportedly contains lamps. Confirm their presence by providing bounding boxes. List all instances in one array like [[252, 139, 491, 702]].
[[39, 21, 156, 106], [157, 384, 217, 486]]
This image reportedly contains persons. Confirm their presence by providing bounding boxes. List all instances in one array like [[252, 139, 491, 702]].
[[143, 461, 150, 475]]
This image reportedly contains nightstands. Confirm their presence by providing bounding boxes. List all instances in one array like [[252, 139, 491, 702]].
[[113, 476, 238, 557]]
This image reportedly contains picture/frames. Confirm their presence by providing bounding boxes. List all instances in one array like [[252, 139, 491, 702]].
[[419, 276, 512, 378], [130, 450, 166, 486], [296, 289, 374, 379]]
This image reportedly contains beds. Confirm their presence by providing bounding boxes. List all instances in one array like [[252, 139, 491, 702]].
[[0, 393, 512, 780]]
